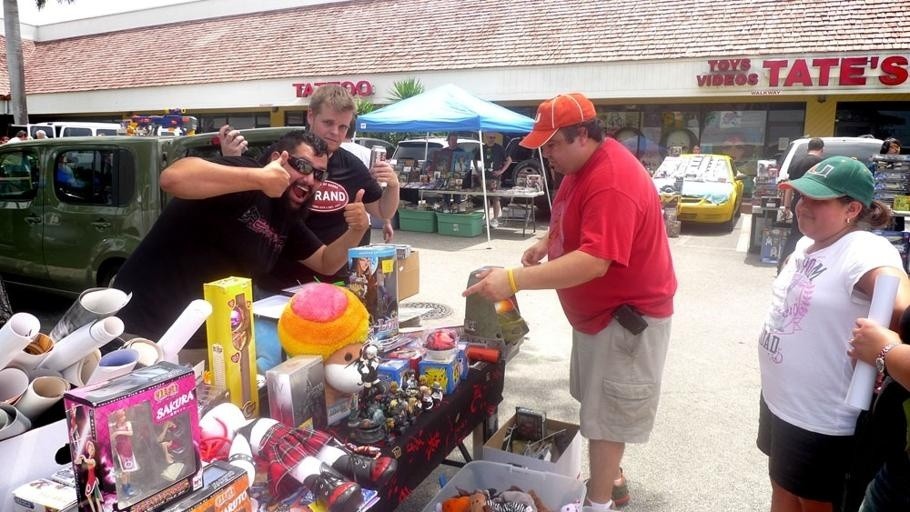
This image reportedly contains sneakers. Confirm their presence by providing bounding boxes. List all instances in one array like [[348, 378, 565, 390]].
[[586, 467, 629, 506]]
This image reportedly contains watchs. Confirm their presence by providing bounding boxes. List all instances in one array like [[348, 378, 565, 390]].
[[875, 342, 893, 373]]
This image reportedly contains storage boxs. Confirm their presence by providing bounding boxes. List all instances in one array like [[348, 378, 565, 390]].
[[399, 205, 438, 233], [419, 460, 586, 511], [484, 415, 586, 485], [435, 211, 483, 237]]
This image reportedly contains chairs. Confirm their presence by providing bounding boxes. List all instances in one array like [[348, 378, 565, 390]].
[[505, 185, 538, 228]]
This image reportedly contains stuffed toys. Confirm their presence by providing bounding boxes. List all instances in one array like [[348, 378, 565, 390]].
[[435, 485, 557, 512], [277, 281, 379, 409]]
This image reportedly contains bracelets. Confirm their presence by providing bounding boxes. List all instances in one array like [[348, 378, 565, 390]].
[[507, 267, 517, 292], [365, 211, 372, 227]]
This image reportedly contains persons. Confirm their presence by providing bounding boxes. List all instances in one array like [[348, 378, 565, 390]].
[[475, 131, 512, 226], [369, 368, 444, 431], [879, 135, 903, 158], [441, 132, 467, 206], [756, 157, 909, 511], [111, 131, 369, 365], [219, 85, 400, 287], [337, 115, 394, 281], [351, 257, 396, 325], [778, 137, 827, 276], [461, 94, 678, 511], [849, 296, 908, 512], [692, 146, 700, 154], [156, 419, 187, 462], [109, 409, 140, 498], [612, 126, 646, 160], [193, 401, 397, 512], [657, 128, 698, 160], [0, 128, 114, 187], [714, 126, 754, 195]]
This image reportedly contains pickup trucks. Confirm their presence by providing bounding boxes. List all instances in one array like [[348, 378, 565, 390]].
[[1, 126, 324, 322]]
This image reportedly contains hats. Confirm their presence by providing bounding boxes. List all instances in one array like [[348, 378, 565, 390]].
[[778, 155, 874, 208], [519, 91, 596, 149]]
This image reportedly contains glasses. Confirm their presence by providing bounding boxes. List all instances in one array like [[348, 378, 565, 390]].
[[279, 151, 328, 183], [488, 137, 496, 139], [448, 138, 457, 140]]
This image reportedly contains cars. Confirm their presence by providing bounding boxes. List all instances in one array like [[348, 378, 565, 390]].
[[652, 153, 747, 231]]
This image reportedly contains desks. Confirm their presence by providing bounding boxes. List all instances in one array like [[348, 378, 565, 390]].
[[418, 188, 546, 239], [0, 360, 504, 512]]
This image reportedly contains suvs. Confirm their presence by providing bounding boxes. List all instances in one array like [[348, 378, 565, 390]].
[[776, 134, 885, 196], [501, 136, 566, 217]]
